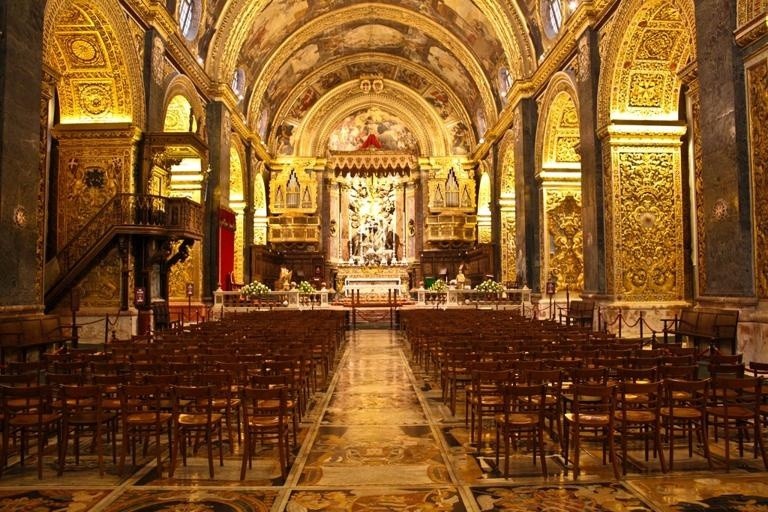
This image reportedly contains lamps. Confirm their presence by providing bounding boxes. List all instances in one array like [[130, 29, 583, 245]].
[[360, 91, 383, 153]]
